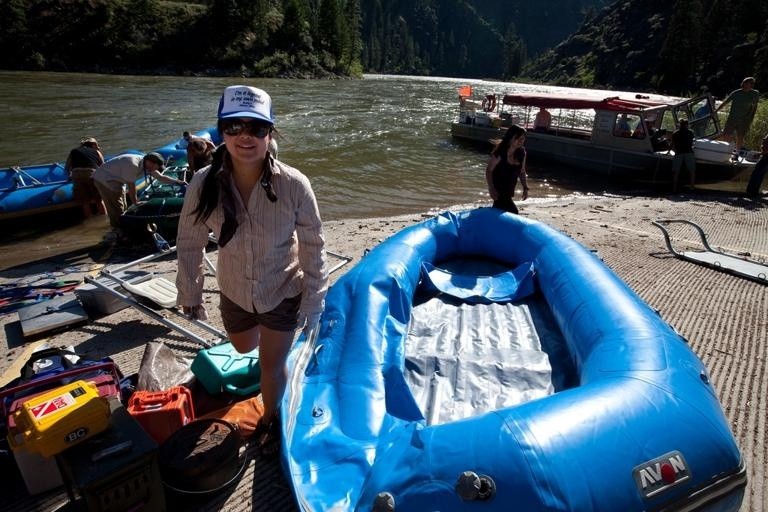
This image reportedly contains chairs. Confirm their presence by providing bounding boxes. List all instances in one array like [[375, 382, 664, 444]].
[[121, 277, 179, 309]]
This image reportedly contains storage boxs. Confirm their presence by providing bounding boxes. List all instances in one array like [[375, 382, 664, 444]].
[[74, 270, 153, 315], [0, 362, 124, 496]]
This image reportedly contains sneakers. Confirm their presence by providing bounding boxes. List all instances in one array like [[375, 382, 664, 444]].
[[254, 416, 280, 456]]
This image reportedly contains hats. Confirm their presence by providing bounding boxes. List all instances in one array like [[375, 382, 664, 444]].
[[146, 151, 166, 170], [217, 84, 275, 125], [81, 138, 101, 149]]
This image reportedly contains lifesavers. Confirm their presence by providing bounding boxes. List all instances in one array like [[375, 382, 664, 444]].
[[482, 94, 497, 111]]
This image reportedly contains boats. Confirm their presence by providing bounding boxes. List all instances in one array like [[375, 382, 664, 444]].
[[119, 122, 227, 249], [0, 147, 143, 224], [281, 206, 748, 512], [449, 86, 758, 187]]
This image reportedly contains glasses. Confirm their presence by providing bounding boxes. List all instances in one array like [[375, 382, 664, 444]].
[[219, 117, 270, 139]]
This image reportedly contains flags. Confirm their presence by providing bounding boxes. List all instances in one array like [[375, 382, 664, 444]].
[[459, 85, 470, 97]]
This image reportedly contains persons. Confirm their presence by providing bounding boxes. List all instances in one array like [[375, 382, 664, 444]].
[[665, 118, 697, 188], [64, 136, 104, 218], [484, 125, 530, 216], [176, 130, 198, 148], [174, 84, 327, 460], [636, 114, 656, 137], [535, 105, 551, 131], [618, 114, 631, 131], [715, 76, 759, 160], [694, 96, 717, 136], [92, 151, 188, 228], [746, 134, 768, 198], [187, 139, 217, 178]]
